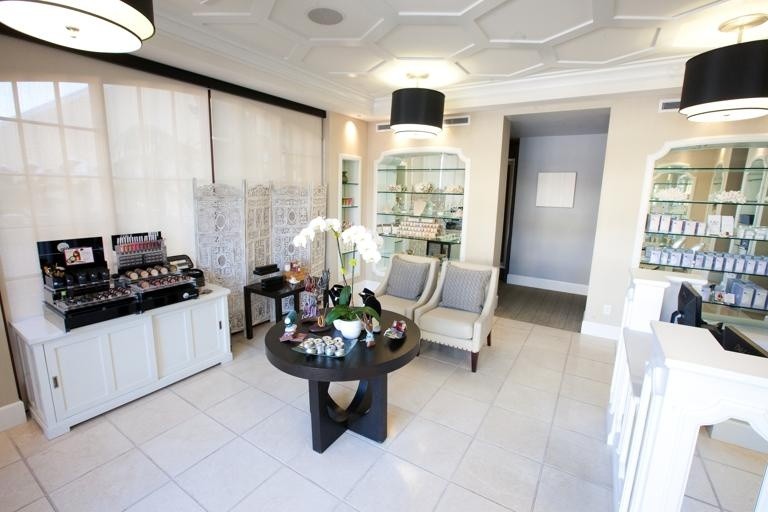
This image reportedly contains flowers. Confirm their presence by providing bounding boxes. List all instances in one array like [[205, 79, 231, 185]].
[[293, 215, 381, 325]]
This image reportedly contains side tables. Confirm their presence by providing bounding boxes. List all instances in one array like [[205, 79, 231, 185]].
[[243, 275, 330, 339]]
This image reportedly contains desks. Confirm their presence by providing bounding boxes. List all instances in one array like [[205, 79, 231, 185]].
[[608, 321, 768, 510], [10, 281, 233, 439], [264, 307, 422, 453]]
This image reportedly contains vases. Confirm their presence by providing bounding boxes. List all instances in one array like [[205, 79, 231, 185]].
[[339, 319, 363, 339]]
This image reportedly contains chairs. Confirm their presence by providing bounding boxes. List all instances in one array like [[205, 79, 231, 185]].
[[415, 261, 501, 372], [374, 254, 439, 320]]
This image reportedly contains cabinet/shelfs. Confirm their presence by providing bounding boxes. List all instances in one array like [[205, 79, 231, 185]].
[[632, 133, 768, 313], [373, 148, 471, 264], [338, 154, 363, 281]]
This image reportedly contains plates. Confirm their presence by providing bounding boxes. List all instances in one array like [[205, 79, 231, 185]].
[[291, 337, 359, 359]]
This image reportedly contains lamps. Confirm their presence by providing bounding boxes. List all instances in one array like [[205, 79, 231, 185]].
[[389, 87, 446, 140], [3, 0, 157, 55], [678, 39, 767, 124]]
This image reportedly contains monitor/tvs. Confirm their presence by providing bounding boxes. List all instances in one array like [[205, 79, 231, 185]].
[[723, 325, 768, 358], [670, 280, 703, 328]]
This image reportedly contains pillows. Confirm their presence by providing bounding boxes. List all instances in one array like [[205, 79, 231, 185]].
[[439, 266, 491, 313], [385, 255, 431, 300]]
